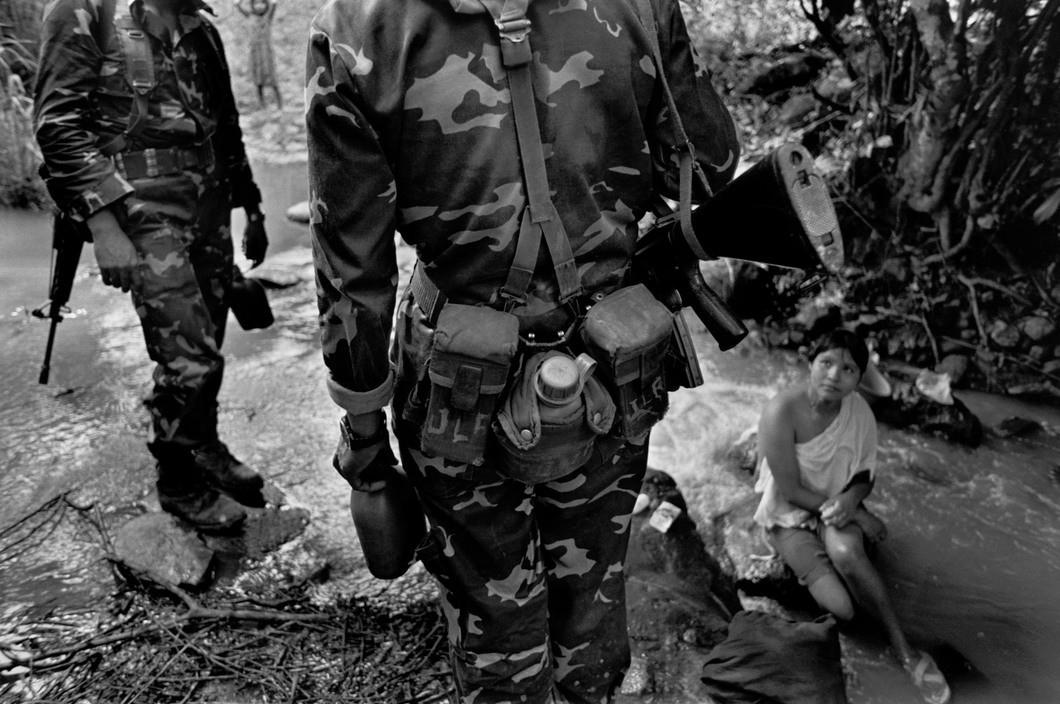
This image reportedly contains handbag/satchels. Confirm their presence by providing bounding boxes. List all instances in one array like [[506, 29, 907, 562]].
[[702, 610, 850, 704]]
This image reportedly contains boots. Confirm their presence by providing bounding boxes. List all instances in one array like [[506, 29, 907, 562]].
[[192, 400, 264, 493], [156, 456, 246, 532]]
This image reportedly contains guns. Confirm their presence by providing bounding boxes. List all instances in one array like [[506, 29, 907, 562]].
[[622, 139, 847, 388], [30, 206, 94, 385]]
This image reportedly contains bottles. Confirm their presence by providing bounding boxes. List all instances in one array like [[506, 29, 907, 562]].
[[533, 352, 598, 426]]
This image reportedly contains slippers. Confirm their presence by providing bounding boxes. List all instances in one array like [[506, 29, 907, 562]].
[[903, 650, 950, 704]]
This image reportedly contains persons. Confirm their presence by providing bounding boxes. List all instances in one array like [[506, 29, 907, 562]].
[[32, 0, 266, 532], [308, 0, 741, 704], [236, 1, 284, 111], [753, 328, 952, 704]]
